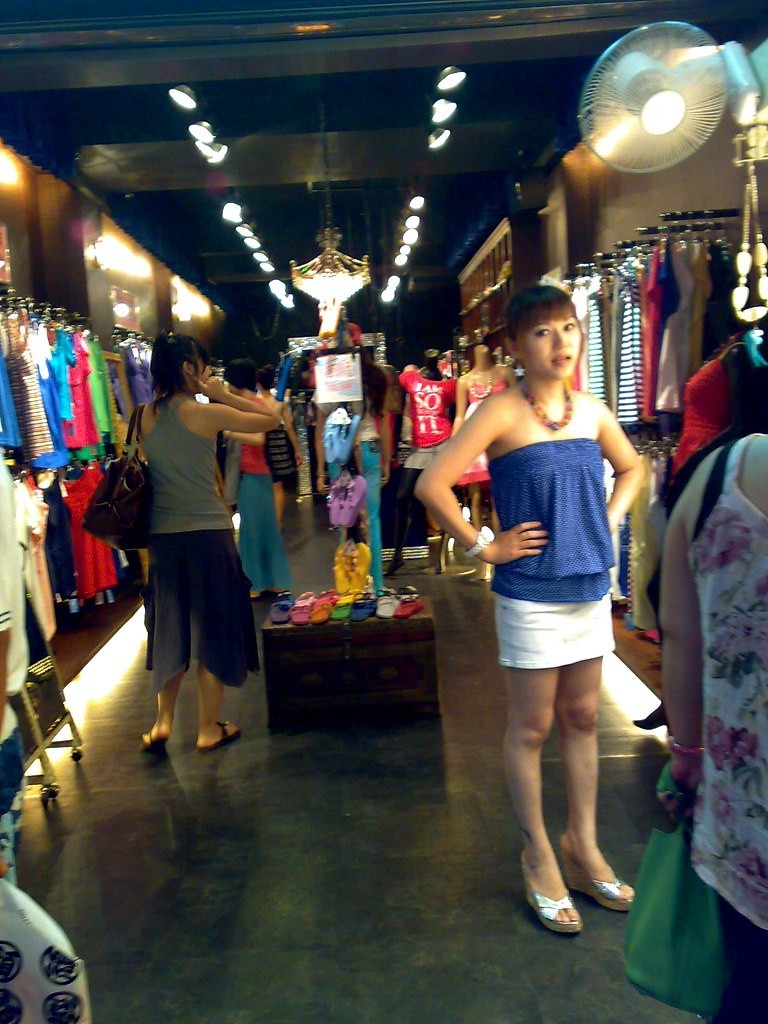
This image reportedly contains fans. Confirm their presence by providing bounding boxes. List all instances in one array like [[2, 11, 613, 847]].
[[579, 21, 768, 323]]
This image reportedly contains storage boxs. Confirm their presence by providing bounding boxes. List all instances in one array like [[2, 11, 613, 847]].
[[260, 593, 442, 731]]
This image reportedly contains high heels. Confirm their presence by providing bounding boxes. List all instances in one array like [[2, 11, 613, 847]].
[[561, 853, 634, 912], [521, 865, 583, 933]]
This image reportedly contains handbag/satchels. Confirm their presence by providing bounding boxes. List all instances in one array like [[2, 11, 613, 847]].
[[622, 764, 722, 1017], [80, 404, 154, 549]]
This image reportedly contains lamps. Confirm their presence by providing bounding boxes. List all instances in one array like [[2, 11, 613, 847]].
[[290, 79, 371, 335], [428, 63, 468, 149], [170, 81, 297, 310]]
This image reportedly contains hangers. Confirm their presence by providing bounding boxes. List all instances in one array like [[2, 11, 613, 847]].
[[575, 210, 731, 273], [719, 317, 765, 361]]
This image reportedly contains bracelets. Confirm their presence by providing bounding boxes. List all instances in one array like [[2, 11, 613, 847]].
[[316, 471, 326, 478]]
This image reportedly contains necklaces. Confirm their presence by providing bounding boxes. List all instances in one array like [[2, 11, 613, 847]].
[[469, 368, 493, 399], [518, 378, 573, 431]]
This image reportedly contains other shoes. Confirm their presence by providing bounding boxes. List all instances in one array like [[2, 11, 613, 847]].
[[270, 587, 287, 593], [249, 592, 260, 599]]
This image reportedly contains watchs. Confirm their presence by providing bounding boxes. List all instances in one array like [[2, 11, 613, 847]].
[[463, 526, 495, 560]]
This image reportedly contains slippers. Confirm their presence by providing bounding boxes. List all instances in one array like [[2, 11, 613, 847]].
[[329, 475, 368, 526], [321, 414, 360, 465], [143, 731, 170, 750], [205, 721, 241, 751], [270, 540, 423, 625]]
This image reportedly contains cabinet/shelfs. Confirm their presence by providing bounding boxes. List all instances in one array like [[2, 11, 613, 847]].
[[457, 212, 542, 369]]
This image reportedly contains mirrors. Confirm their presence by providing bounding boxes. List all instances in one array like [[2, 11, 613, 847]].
[[100, 350, 149, 583]]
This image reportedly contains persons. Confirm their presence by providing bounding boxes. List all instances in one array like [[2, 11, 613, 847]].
[[0, 447, 29, 813], [310, 321, 393, 592], [661, 432, 768, 1024], [133, 327, 303, 748], [382, 349, 459, 579], [451, 344, 519, 580], [418, 285, 645, 935]]
